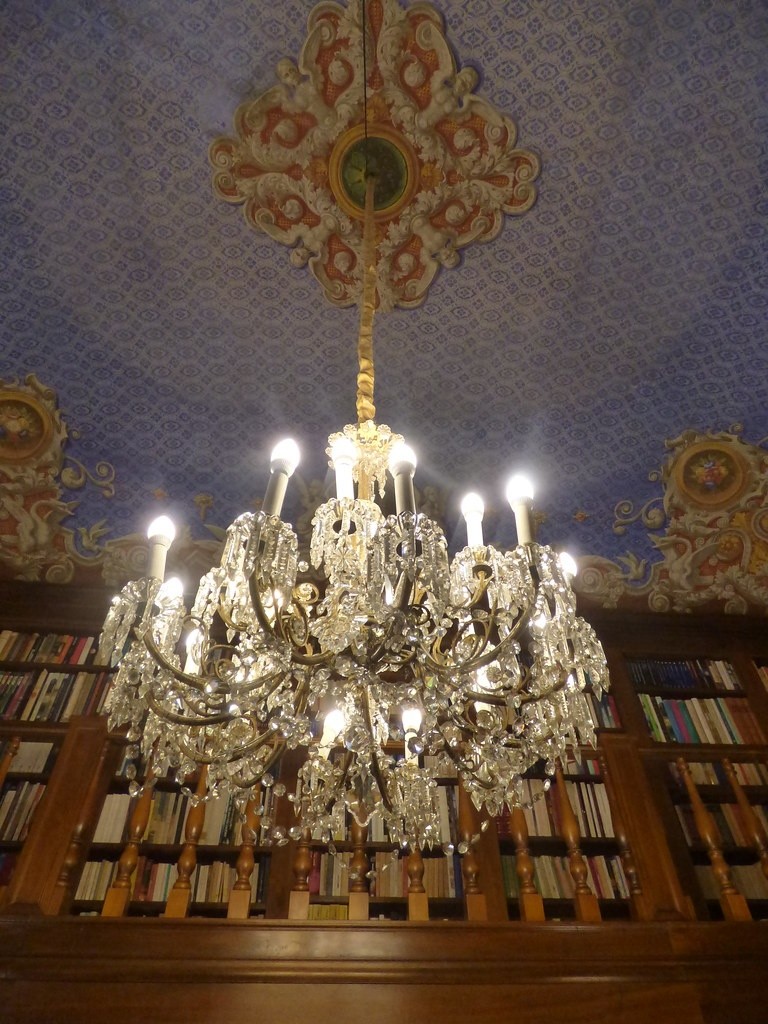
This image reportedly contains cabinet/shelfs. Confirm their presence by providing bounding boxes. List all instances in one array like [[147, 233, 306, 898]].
[[0, 575, 768, 1023]]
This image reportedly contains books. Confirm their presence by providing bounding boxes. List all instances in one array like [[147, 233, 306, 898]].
[[71, 745, 282, 918], [499, 651, 631, 899], [0, 630, 131, 842], [310, 718, 459, 920], [627, 655, 768, 901]]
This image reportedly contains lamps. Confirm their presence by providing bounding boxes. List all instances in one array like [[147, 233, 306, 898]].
[[102, 187, 609, 887]]
